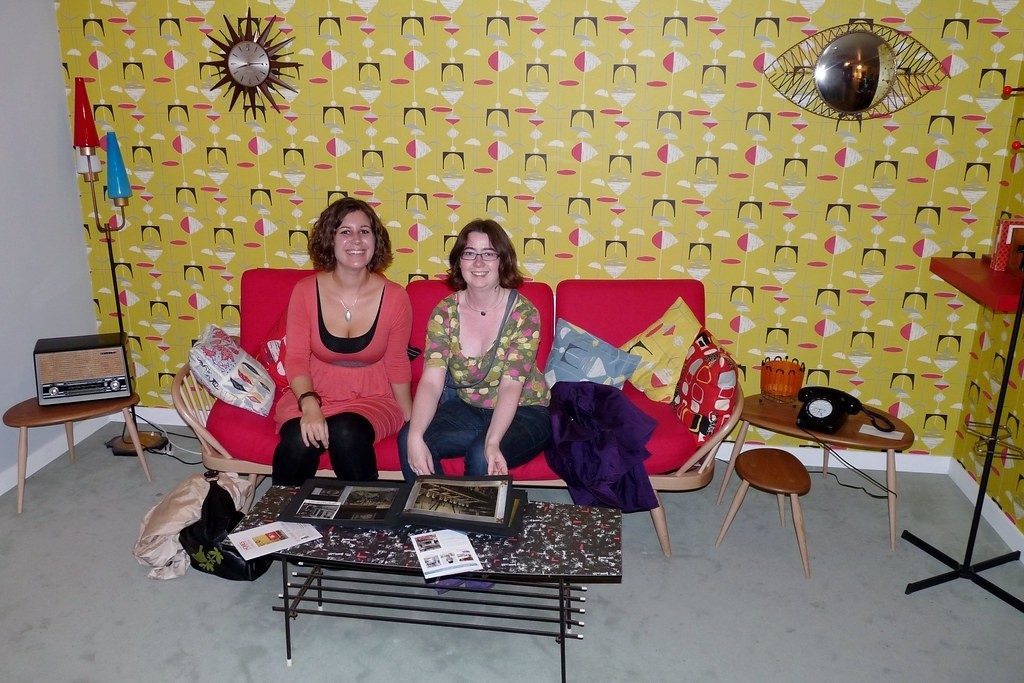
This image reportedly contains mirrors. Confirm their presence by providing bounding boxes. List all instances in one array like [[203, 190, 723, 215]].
[[764, 22, 950, 122]]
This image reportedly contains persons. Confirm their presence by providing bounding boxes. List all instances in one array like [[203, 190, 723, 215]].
[[272, 197, 413, 487], [396, 219, 551, 486]]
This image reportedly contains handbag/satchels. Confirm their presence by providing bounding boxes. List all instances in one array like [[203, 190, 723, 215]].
[[178, 471, 273, 581]]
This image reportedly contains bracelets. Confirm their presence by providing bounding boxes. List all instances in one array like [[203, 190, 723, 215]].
[[298, 391, 322, 411]]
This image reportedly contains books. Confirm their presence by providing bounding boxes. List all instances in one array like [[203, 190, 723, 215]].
[[989, 219, 1024, 271]]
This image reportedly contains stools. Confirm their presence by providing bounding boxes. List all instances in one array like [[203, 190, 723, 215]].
[[714, 447, 812, 578]]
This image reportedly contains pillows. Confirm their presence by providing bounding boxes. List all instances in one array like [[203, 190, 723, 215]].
[[617, 296, 741, 406], [407, 305, 432, 384], [249, 306, 292, 395], [189, 324, 277, 418], [543, 316, 642, 393], [669, 326, 739, 450]]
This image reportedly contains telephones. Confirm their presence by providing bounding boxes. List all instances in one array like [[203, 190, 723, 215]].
[[798, 386, 863, 433]]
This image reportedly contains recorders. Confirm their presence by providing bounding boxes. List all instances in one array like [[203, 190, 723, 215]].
[[33, 332, 133, 406]]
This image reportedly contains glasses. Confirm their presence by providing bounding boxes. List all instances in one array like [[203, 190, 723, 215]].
[[461, 251, 500, 261]]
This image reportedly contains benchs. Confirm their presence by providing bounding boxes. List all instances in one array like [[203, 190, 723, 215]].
[[169, 269, 744, 558]]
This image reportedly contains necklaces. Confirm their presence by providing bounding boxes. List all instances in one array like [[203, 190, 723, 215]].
[[465, 288, 505, 316], [339, 296, 357, 322]]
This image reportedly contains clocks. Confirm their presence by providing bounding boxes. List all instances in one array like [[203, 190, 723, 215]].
[[200, 4, 304, 124]]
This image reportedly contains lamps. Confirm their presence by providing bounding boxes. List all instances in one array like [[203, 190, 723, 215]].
[[70, 76, 168, 456]]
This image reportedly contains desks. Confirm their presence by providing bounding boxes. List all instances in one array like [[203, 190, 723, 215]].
[[901, 253, 1024, 613], [3, 393, 153, 514], [220, 485, 624, 683], [717, 391, 914, 552]]
